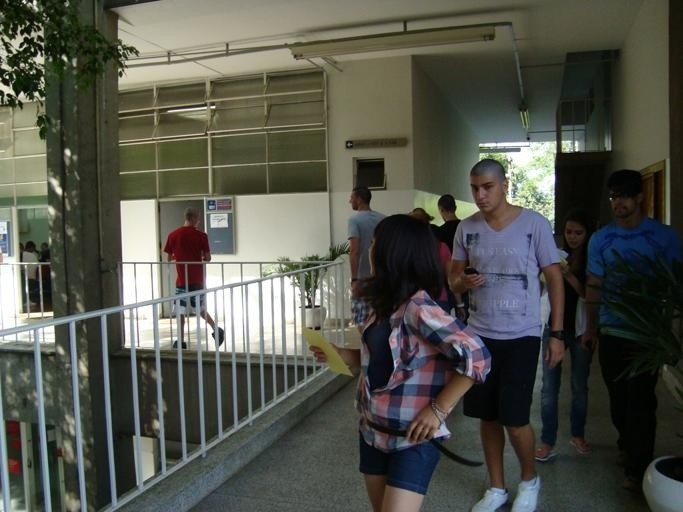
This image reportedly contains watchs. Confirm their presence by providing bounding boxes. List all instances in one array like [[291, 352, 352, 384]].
[[549, 330, 565, 340]]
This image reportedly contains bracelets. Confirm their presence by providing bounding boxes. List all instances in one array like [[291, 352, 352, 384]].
[[431, 398, 448, 424], [456, 303, 465, 308]]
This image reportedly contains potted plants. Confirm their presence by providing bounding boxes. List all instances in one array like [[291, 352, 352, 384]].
[[269, 242, 353, 327], [588, 244, 681, 512]]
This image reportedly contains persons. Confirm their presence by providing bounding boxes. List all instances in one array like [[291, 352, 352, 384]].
[[309, 214, 495, 511], [18, 239, 51, 307], [162, 207, 224, 349], [346, 185, 388, 335], [582, 169, 682, 490], [411, 208, 466, 321], [535, 210, 599, 463], [429, 194, 462, 256], [447, 158, 568, 512]]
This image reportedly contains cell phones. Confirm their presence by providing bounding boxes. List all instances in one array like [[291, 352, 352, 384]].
[[464, 268, 480, 275]]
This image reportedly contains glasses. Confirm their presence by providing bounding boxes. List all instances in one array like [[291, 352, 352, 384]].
[[608, 193, 627, 203]]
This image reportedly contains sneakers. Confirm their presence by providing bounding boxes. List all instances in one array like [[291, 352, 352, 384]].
[[614, 445, 640, 490], [511, 473, 540, 512], [570, 436, 592, 455], [212, 328, 224, 346], [472, 488, 509, 512], [534, 444, 557, 461], [173, 341, 186, 349]]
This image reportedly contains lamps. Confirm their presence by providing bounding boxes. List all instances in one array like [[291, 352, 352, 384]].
[[289, 24, 496, 61], [516, 100, 530, 130]]
